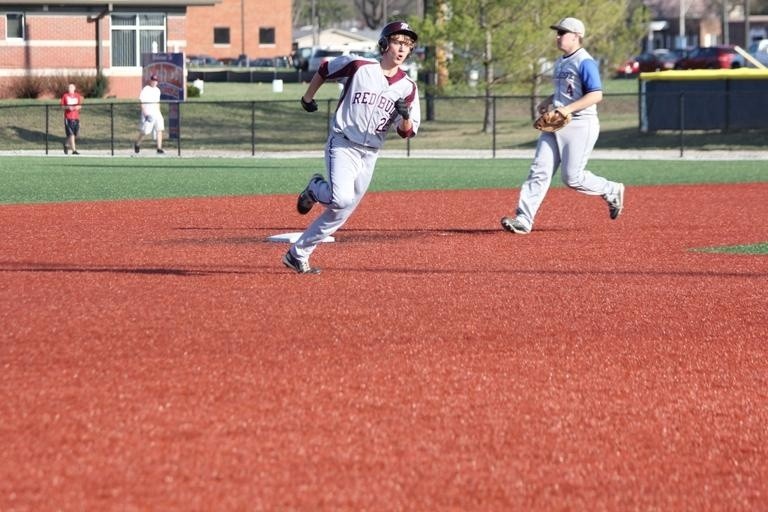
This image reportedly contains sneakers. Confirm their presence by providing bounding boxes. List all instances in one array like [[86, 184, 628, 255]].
[[604, 181, 624, 220], [283, 250, 322, 275], [60, 144, 167, 155], [297, 173, 324, 215], [501, 216, 532, 235]]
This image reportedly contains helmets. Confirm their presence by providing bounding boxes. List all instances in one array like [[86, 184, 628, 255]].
[[381, 21, 418, 44]]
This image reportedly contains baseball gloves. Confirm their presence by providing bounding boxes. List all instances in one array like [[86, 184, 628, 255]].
[[533, 108, 572, 132]]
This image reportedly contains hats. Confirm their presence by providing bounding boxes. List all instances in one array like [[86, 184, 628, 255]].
[[549, 17, 586, 36], [150, 74, 159, 81]]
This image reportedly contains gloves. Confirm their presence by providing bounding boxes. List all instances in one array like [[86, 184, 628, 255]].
[[299, 96, 319, 112], [395, 97, 410, 119]]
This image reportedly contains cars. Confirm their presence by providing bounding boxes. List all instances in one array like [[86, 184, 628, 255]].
[[183, 46, 425, 71], [611, 38, 768, 79]]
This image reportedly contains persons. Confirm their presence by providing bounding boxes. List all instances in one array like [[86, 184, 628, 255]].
[[502, 16, 625, 235], [134, 75, 168, 153], [283, 22, 422, 275], [60, 81, 83, 153]]
